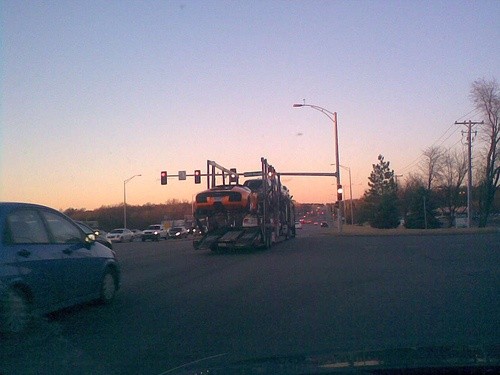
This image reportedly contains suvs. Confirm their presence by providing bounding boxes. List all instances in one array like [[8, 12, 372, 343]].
[[140, 224, 169, 242]]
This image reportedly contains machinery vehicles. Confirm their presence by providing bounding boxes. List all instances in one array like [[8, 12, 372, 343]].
[[192, 156, 296, 249]]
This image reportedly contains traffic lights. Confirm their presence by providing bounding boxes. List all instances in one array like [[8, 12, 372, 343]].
[[194, 170, 201, 184], [161, 171, 167, 186], [268, 166, 275, 180], [229, 168, 237, 182]]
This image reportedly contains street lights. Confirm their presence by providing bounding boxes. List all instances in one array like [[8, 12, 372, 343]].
[[292, 102, 343, 234], [330, 164, 354, 226], [124, 174, 142, 229]]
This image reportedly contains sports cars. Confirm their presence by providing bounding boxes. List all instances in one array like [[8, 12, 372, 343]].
[[196, 184, 258, 212]]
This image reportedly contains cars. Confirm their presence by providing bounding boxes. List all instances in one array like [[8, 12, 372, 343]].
[[187, 226, 208, 235], [0, 201, 122, 322], [131, 229, 141, 238], [27, 220, 113, 250], [105, 228, 135, 243], [295, 211, 329, 229], [168, 227, 189, 239]]
